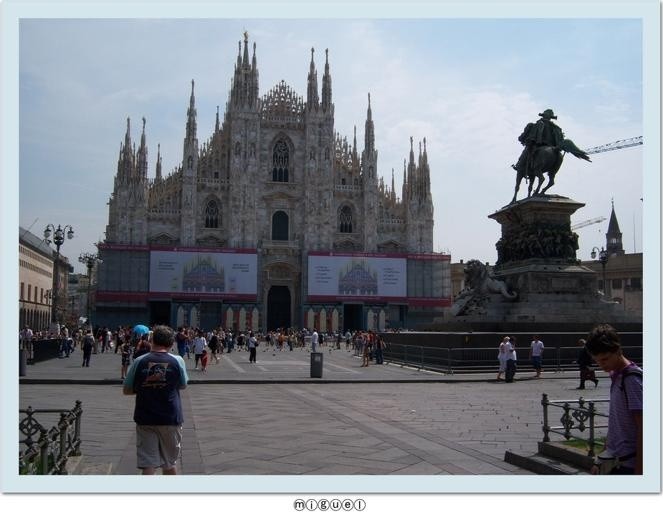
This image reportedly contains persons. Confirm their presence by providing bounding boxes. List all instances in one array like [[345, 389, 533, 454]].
[[497, 337, 509, 380], [528, 335, 545, 381], [518, 109, 566, 147], [123, 324, 188, 475], [503, 335, 518, 384], [576, 338, 599, 391], [588, 325, 643, 475], [19, 321, 388, 378]]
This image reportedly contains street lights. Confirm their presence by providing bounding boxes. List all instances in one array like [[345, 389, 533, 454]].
[[591, 247, 608, 293], [78, 252, 104, 325], [44, 224, 74, 335]]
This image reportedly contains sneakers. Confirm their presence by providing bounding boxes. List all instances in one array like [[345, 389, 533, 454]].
[[576, 386, 586, 390], [496, 377, 515, 383], [595, 379, 599, 387]]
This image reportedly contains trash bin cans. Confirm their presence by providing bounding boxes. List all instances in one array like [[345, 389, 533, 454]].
[[311, 353, 322, 378], [19, 350, 26, 376]]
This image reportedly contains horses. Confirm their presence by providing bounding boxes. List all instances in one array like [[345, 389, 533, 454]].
[[509, 123, 592, 205]]
